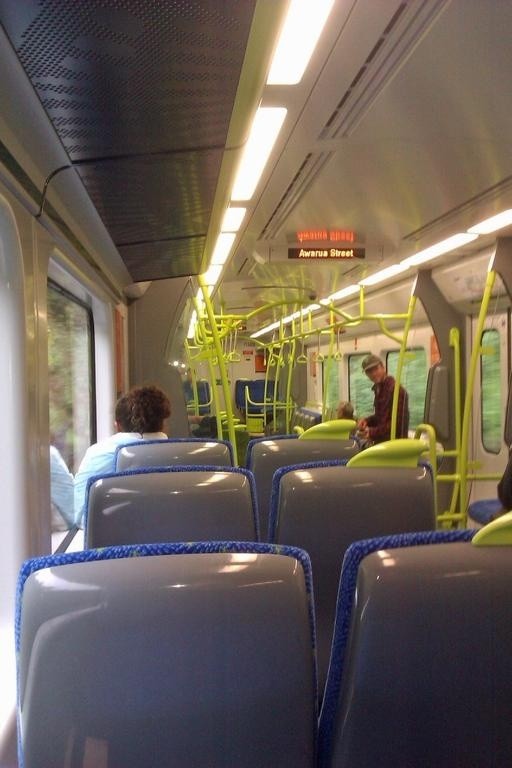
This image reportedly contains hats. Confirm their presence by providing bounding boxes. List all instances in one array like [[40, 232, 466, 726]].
[[362, 355, 381, 372]]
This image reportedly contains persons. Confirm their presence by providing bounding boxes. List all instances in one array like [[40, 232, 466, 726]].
[[49, 445, 73, 530], [187, 415, 218, 438], [74, 387, 170, 530], [183, 368, 197, 401], [336, 401, 354, 419], [358, 355, 409, 445]]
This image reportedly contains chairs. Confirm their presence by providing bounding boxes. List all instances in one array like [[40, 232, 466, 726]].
[[10, 422, 511, 760]]
